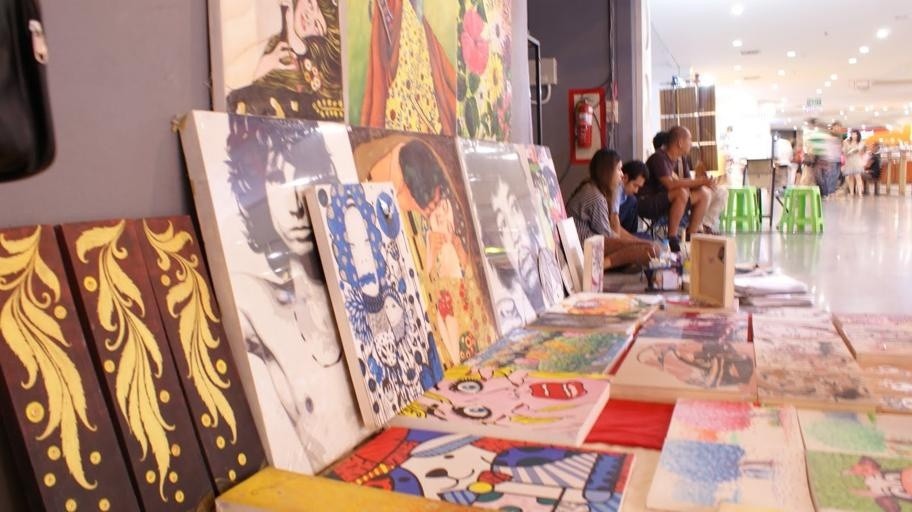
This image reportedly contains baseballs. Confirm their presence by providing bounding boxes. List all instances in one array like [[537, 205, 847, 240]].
[[574, 97, 593, 147]]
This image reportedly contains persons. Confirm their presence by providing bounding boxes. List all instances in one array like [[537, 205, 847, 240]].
[[566, 125, 717, 272], [771, 118, 881, 199]]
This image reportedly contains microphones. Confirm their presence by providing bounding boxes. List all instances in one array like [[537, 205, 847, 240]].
[[864, 179, 880, 195], [638, 216, 690, 240], [721, 186, 761, 235], [779, 185, 825, 234]]
